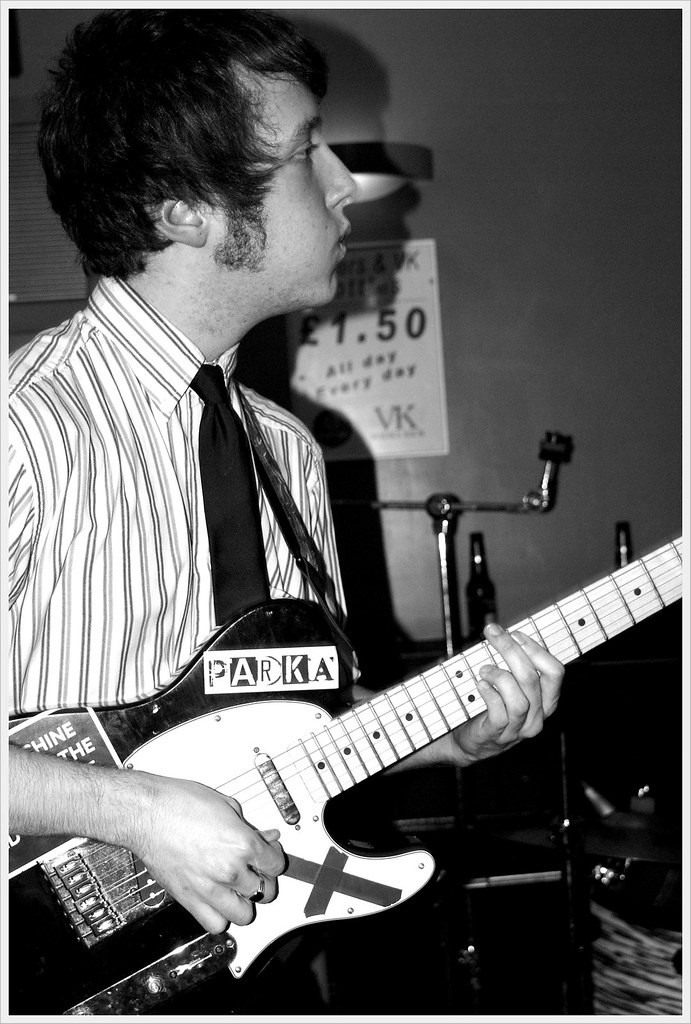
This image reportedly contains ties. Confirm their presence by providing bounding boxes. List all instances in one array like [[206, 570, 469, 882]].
[[189, 366, 275, 627]]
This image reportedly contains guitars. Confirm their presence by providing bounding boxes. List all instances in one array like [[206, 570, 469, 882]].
[[10, 532, 681, 1014]]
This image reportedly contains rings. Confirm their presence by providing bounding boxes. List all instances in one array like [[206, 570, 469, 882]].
[[247, 876, 264, 904]]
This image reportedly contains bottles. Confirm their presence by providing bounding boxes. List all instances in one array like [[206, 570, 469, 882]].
[[466, 531, 497, 641], [615, 521, 632, 568]]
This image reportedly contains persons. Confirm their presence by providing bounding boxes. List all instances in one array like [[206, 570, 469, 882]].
[[9, 9, 566, 1014]]
